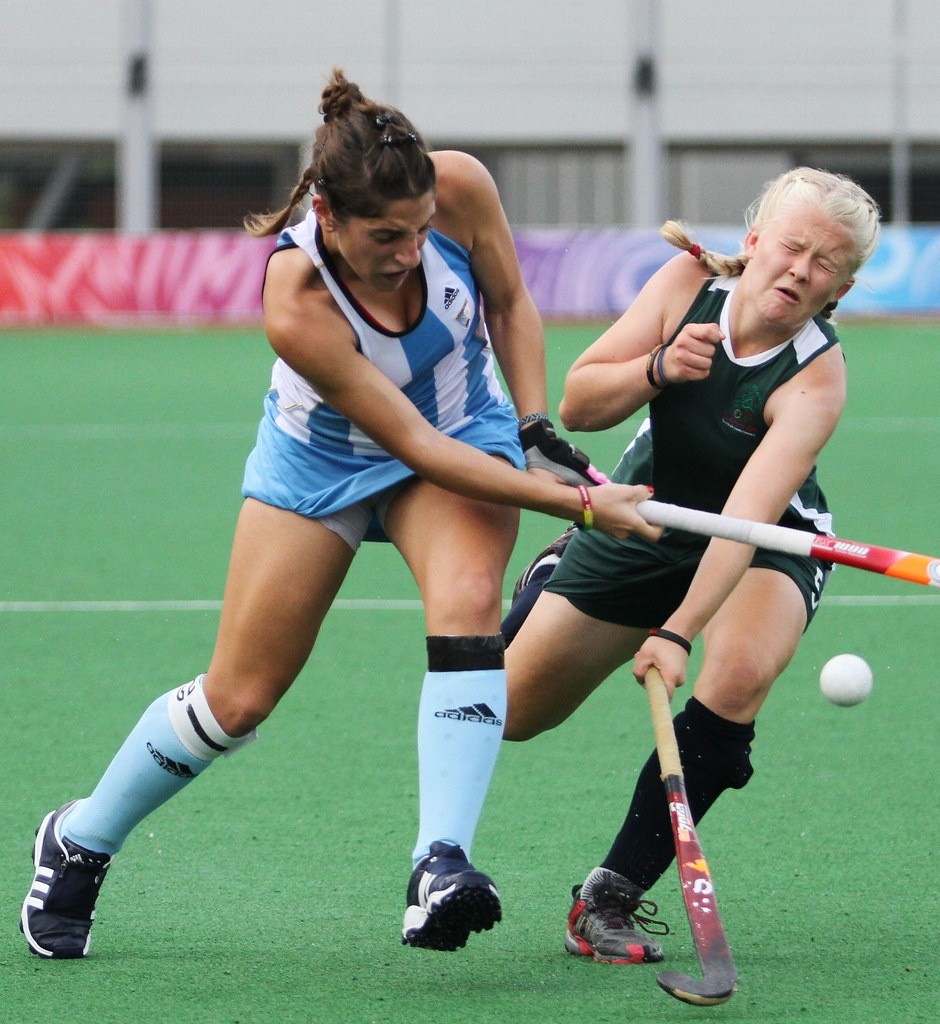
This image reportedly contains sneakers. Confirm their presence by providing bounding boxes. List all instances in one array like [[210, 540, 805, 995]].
[[564, 867, 670, 964], [511, 522, 579, 604], [401, 841, 502, 951], [19, 800, 114, 958]]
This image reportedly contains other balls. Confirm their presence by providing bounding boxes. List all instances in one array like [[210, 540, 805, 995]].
[[819, 654, 873, 708]]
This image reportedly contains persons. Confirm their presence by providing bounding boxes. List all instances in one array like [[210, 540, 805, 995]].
[[17, 68, 663, 959], [502, 168, 881, 964]]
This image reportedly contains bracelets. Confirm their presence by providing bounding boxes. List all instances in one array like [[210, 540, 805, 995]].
[[576, 484, 593, 535], [648, 629, 692, 656], [647, 343, 673, 391]]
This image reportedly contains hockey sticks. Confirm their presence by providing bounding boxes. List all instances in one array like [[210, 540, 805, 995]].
[[635, 500, 940, 588], [642, 664, 739, 1006]]
